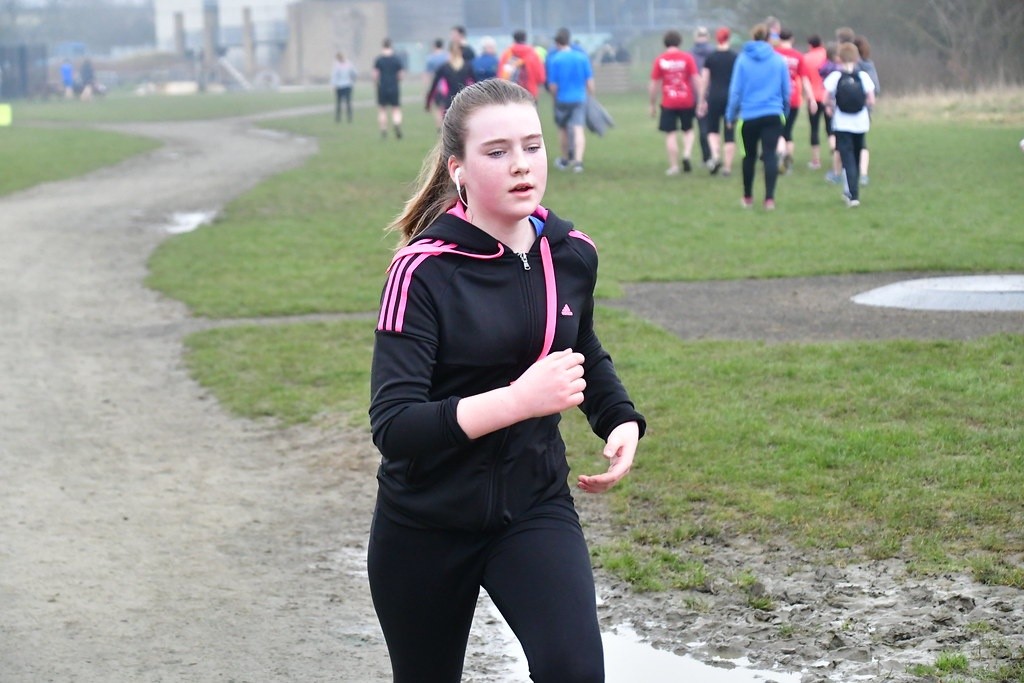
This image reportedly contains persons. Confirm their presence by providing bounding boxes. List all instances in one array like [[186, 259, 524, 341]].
[[368, 37, 409, 140], [331, 52, 355, 124], [425, 16, 882, 210], [74, 51, 101, 96], [59, 57, 77, 98], [367, 77, 644, 682]]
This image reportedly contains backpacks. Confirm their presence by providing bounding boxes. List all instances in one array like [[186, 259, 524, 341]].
[[834, 69, 866, 113]]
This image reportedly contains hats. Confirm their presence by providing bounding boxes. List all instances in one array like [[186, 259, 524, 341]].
[[692, 26, 712, 45]]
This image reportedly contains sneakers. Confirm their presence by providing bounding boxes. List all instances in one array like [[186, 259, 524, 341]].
[[682, 157, 692, 172], [842, 191, 860, 208], [807, 161, 821, 170], [722, 169, 732, 177], [573, 162, 584, 173], [763, 198, 776, 210], [710, 159, 722, 176], [555, 157, 569, 170], [741, 197, 753, 209], [858, 173, 869, 187], [665, 167, 679, 177], [826, 172, 843, 184]]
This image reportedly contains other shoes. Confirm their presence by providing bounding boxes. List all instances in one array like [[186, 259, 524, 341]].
[[382, 130, 388, 138], [777, 155, 793, 174], [394, 124, 403, 138]]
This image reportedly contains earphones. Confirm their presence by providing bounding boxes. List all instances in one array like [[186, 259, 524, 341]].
[[454, 167, 463, 190]]
